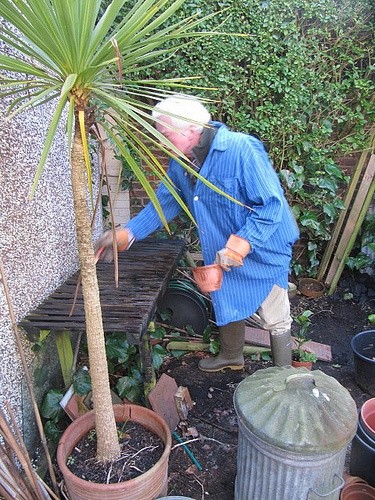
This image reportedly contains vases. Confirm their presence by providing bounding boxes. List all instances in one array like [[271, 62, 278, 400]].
[[350, 397, 375, 488], [351, 329, 375, 392], [340, 473, 375, 500]]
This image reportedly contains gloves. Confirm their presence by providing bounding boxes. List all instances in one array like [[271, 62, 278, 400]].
[[214, 234, 251, 271], [95, 226, 135, 263]]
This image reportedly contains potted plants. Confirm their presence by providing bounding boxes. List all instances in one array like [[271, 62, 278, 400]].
[[0, 0, 257, 500]]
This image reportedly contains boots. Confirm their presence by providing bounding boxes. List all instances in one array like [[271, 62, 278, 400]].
[[198, 320, 245, 372], [269, 329, 291, 366]]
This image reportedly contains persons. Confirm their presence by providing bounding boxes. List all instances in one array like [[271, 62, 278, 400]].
[[94, 93, 299, 372]]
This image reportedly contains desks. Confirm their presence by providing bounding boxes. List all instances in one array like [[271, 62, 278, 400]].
[[18, 240, 185, 410]]
